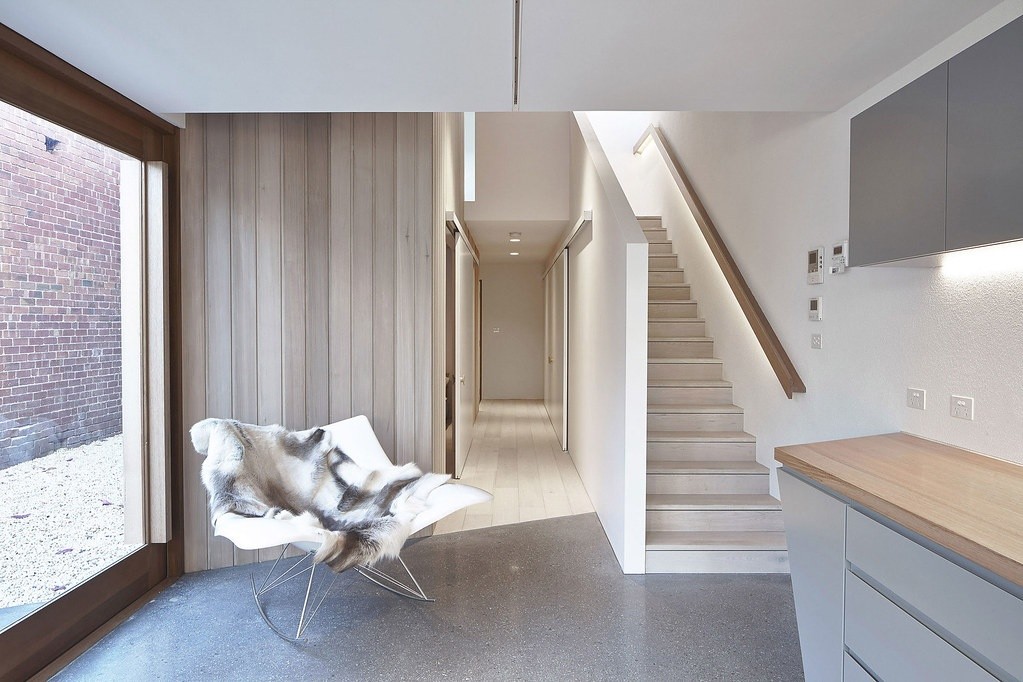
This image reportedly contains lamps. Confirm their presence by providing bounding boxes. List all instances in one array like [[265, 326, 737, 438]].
[[509, 249, 521, 256], [46, 135, 63, 152], [509, 233, 521, 242]]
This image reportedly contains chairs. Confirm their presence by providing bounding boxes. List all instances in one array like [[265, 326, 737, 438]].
[[189, 415, 494, 644]]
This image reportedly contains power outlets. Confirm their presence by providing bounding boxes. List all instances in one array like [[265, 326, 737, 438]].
[[907, 388, 925, 411], [950, 396, 973, 421]]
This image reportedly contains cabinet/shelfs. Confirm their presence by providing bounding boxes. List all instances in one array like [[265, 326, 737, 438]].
[[849, 14, 1023, 272], [774, 431, 1023, 682]]
